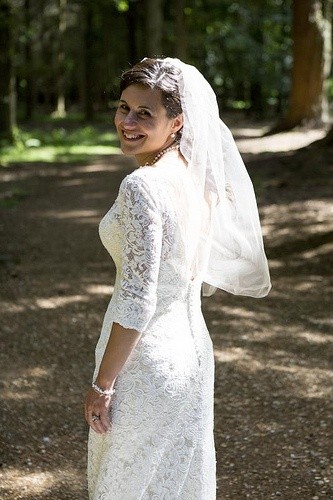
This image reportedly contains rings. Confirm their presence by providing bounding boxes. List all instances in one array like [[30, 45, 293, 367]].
[[91, 414, 100, 423]]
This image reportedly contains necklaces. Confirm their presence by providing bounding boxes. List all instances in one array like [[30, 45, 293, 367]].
[[149, 145, 176, 165]]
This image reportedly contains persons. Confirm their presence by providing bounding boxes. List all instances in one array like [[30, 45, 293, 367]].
[[84, 56, 271, 500]]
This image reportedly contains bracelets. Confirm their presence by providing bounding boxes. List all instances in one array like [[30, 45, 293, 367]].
[[91, 383, 116, 398]]
